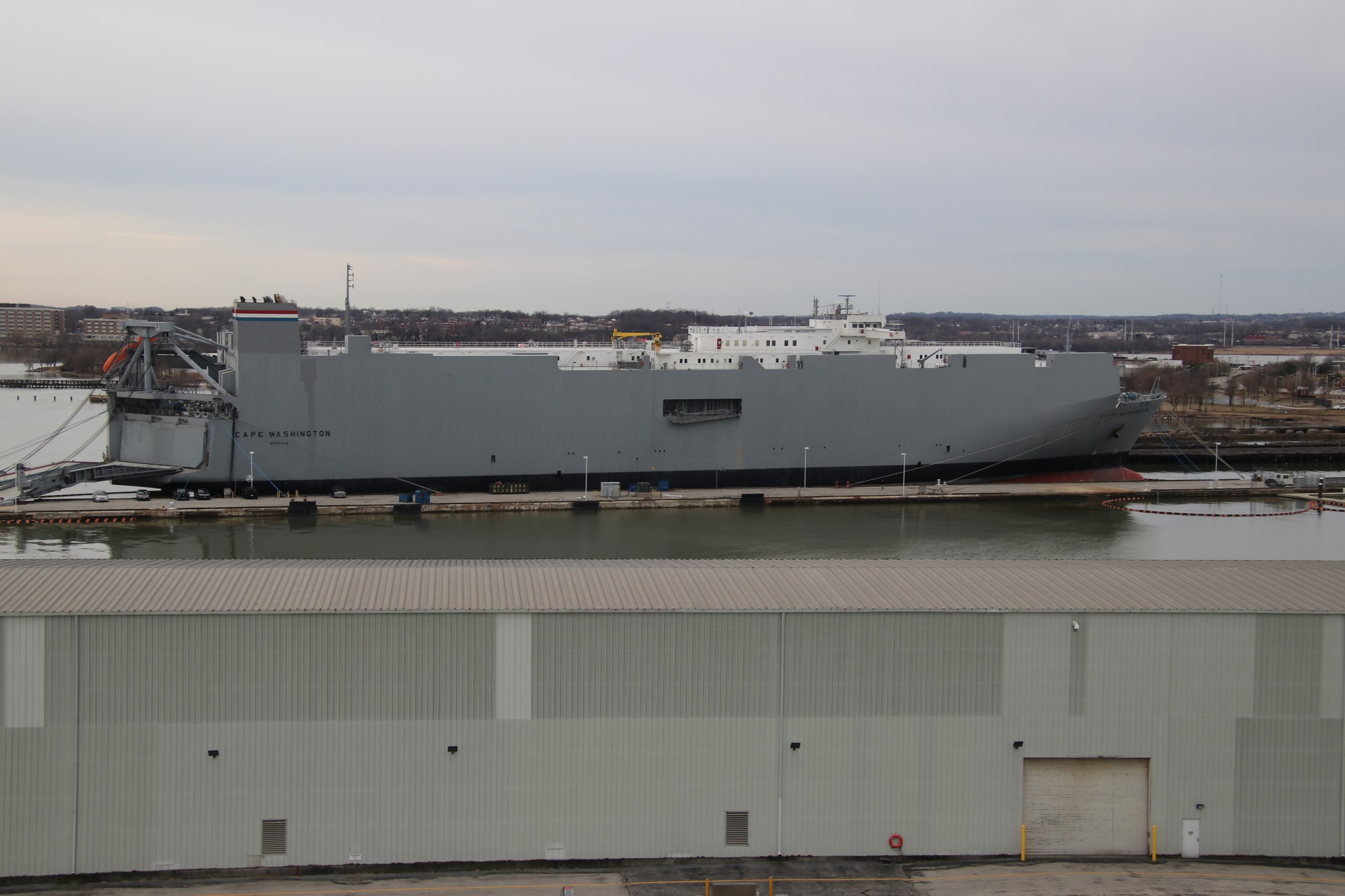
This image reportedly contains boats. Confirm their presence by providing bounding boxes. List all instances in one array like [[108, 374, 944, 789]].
[[103, 336, 160, 375]]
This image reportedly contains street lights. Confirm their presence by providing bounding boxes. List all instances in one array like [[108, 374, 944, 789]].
[[1201, 364, 1207, 373], [1262, 374, 1269, 384], [1215, 443, 1221, 481], [249, 451, 254, 488], [1188, 367, 1194, 380], [1333, 377, 1340, 402], [1185, 365, 1190, 380], [1182, 369, 1188, 382], [804, 447, 809, 489], [1275, 376, 1282, 390], [1173, 372, 1180, 384], [1314, 364, 1340, 379], [901, 453, 906, 496], [1206, 363, 1214, 379], [583, 456, 588, 501]]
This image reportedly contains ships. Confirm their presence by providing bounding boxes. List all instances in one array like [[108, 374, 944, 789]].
[[14, 263, 1170, 504]]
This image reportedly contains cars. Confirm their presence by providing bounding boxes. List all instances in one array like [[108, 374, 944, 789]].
[[173, 488, 190, 501], [242, 487, 258, 500], [330, 486, 346, 498], [1333, 405, 1345, 410], [1210, 380, 1215, 386], [194, 488, 210, 501], [92, 491, 109, 502], [136, 489, 151, 501]]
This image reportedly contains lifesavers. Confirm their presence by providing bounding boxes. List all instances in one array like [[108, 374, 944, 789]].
[[1114, 361, 1118, 365], [379, 348, 383, 352], [785, 363, 789, 368], [641, 360, 644, 365], [457, 341, 460, 347], [888, 834, 903, 849], [943, 358, 946, 362]]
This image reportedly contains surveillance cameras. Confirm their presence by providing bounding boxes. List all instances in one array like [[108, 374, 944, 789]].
[[1073, 622, 1079, 629]]
